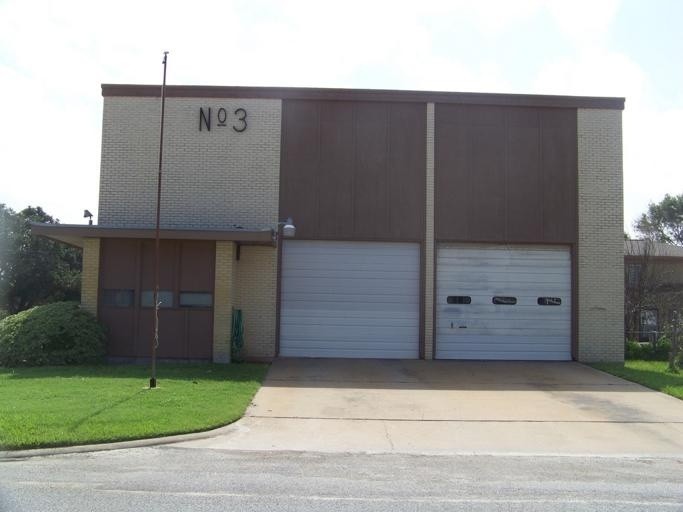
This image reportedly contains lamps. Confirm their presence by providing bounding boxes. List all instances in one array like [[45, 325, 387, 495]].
[[269, 216, 297, 236]]
